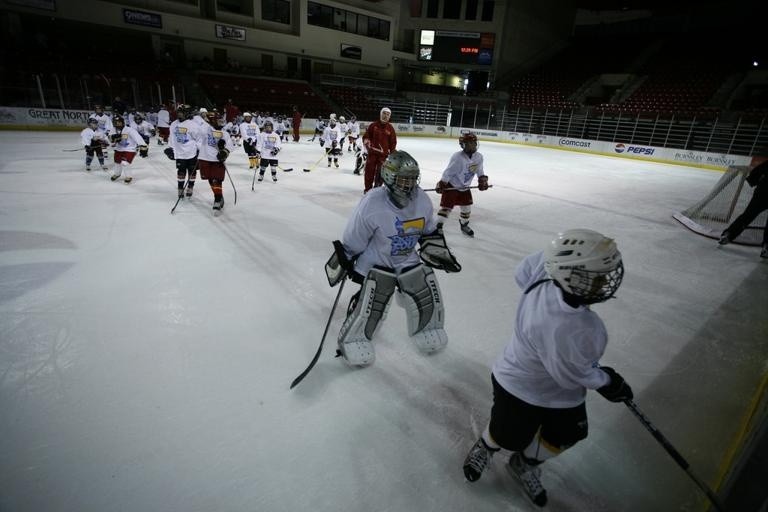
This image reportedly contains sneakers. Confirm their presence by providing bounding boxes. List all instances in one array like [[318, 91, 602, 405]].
[[185, 185, 194, 197], [457, 218, 475, 235], [462, 437, 497, 484], [759, 243, 768, 258], [211, 194, 225, 210], [348, 144, 358, 152], [327, 157, 341, 168], [437, 221, 445, 236], [176, 188, 184, 198], [508, 448, 552, 508], [247, 165, 279, 182], [84, 152, 134, 184], [717, 229, 732, 244]]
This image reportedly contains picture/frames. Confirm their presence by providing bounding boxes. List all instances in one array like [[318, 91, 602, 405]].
[[215, 24, 246, 41], [340, 43, 361, 60], [122, 8, 162, 28], [2, 0, 57, 12]]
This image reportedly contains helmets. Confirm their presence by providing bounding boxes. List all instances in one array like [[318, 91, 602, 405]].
[[84, 96, 359, 130], [541, 227, 624, 302], [459, 132, 477, 150], [379, 150, 420, 198]]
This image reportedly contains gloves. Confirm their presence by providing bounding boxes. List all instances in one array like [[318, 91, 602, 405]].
[[216, 148, 230, 162], [269, 147, 279, 156], [162, 146, 177, 161], [597, 364, 636, 405], [332, 140, 338, 148], [138, 145, 150, 159], [476, 175, 488, 192], [435, 179, 448, 194], [319, 138, 325, 147]]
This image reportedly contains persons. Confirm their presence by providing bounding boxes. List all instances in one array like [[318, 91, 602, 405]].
[[338, 151, 453, 367], [436, 133, 488, 236], [718, 160, 768, 256], [463, 229, 633, 508]]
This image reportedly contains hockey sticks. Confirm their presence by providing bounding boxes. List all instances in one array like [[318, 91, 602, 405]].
[[252, 157, 259, 193], [170, 159, 199, 212], [303, 147, 333, 172], [290, 278, 344, 388], [216, 145, 236, 206], [62, 145, 105, 152], [278, 165, 292, 172]]
